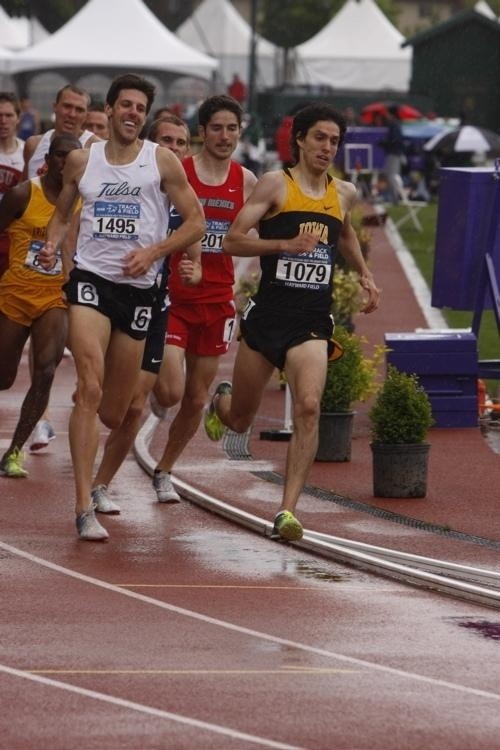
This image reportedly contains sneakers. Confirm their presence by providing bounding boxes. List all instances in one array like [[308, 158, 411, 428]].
[[75, 509, 110, 540], [151, 469, 181, 504], [1, 445, 29, 478], [269, 510, 304, 542], [30, 418, 57, 451], [204, 380, 233, 442], [149, 382, 168, 417], [90, 483, 121, 514]]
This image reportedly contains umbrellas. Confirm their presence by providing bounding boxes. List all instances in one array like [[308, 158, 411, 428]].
[[422, 123, 500, 161], [360, 102, 420, 127]]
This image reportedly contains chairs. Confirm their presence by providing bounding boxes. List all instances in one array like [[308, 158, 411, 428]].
[[390, 174, 430, 234]]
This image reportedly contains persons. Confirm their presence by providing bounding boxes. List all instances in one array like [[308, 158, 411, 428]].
[[211, 104, 382, 541], [0, 135, 83, 480], [341, 104, 362, 128], [88, 116, 203, 513], [375, 104, 411, 205], [20, 83, 102, 450], [148, 94, 262, 503], [1, 70, 193, 180], [38, 76, 207, 544]]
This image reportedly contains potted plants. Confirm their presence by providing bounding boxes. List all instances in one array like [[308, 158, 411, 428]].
[[360, 360, 439, 501], [272, 323, 395, 465]]
